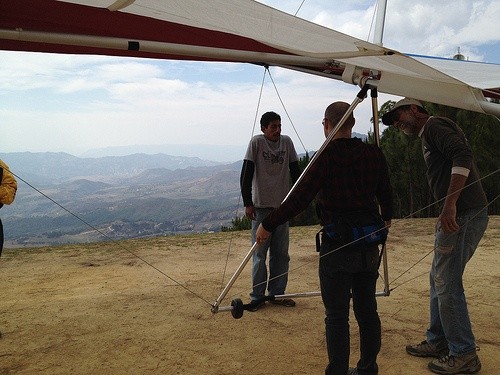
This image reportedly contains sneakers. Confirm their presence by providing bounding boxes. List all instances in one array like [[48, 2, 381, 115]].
[[428, 348, 481, 375], [406, 340, 450, 358], [247, 299, 265, 311], [348, 367, 378, 375], [268, 294, 295, 307]]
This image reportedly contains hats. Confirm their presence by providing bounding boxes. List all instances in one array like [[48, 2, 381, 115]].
[[382, 97, 423, 125]]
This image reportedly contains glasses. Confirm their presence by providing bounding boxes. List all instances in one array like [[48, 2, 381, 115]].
[[389, 106, 410, 124], [322, 122, 325, 126]]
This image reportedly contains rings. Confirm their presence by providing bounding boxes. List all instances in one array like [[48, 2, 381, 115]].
[[448, 224, 452, 227]]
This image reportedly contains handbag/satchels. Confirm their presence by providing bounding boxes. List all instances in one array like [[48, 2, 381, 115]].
[[316, 212, 388, 252]]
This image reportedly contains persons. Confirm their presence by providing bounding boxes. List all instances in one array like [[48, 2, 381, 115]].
[[381, 98, 489, 375], [240, 110, 302, 312], [0, 161, 19, 257], [254, 101, 394, 375]]
